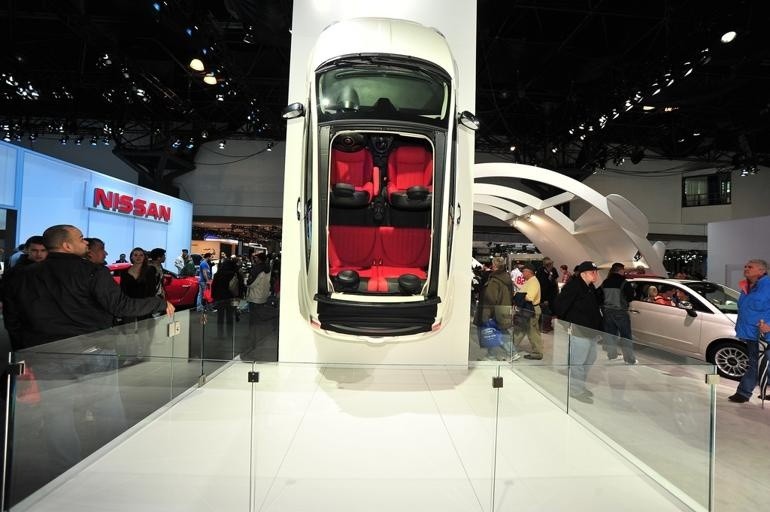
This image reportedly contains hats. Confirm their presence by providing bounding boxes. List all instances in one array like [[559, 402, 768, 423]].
[[518, 261, 537, 271], [577, 260, 600, 273]]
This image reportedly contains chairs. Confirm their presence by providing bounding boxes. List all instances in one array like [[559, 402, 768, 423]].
[[329, 151, 381, 210], [387, 146, 433, 211]]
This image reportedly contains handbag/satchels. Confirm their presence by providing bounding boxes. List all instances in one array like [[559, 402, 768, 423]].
[[479, 317, 503, 348], [228, 273, 239, 296]]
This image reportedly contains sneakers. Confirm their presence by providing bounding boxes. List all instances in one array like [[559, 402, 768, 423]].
[[758, 395, 770, 400], [479, 352, 639, 404], [728, 393, 749, 402]]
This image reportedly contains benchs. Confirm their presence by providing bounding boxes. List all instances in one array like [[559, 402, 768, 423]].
[[328, 225, 431, 296]]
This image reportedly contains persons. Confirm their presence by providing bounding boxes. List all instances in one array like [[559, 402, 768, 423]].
[[728, 259, 770, 403], [0, 225, 281, 360], [473, 253, 689, 402]]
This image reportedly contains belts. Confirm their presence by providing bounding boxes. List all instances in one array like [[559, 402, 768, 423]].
[[533, 304, 538, 306]]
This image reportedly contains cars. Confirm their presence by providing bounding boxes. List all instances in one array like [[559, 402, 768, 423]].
[[106, 263, 216, 309], [281, 13, 484, 349], [624, 272, 760, 381]]
